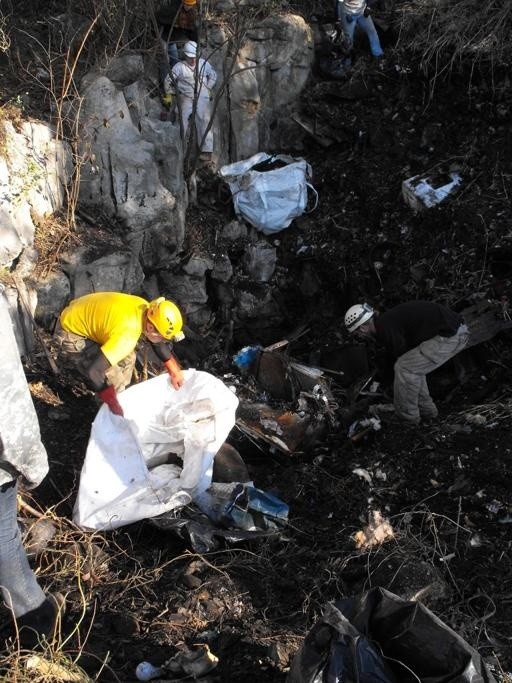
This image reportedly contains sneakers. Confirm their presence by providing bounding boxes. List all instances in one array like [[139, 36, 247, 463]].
[[198, 152, 213, 161], [45, 592, 66, 642], [379, 409, 438, 426]]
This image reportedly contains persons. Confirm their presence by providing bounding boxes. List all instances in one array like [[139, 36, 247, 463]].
[[343, 299, 470, 425], [165, 37, 217, 170], [335, 0, 385, 57], [53, 291, 184, 416], [0, 290, 68, 645]]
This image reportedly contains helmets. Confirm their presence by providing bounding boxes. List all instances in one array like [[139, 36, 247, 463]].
[[345, 302, 376, 334], [146, 297, 185, 343], [183, 0, 197, 6], [183, 40, 200, 58]]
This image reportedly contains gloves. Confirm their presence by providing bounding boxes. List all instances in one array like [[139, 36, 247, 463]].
[[161, 93, 172, 105], [164, 352, 184, 392], [335, 20, 342, 30], [363, 6, 371, 18], [96, 384, 124, 417]]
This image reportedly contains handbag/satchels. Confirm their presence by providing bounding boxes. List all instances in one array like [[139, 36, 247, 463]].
[[218, 150, 320, 236]]
